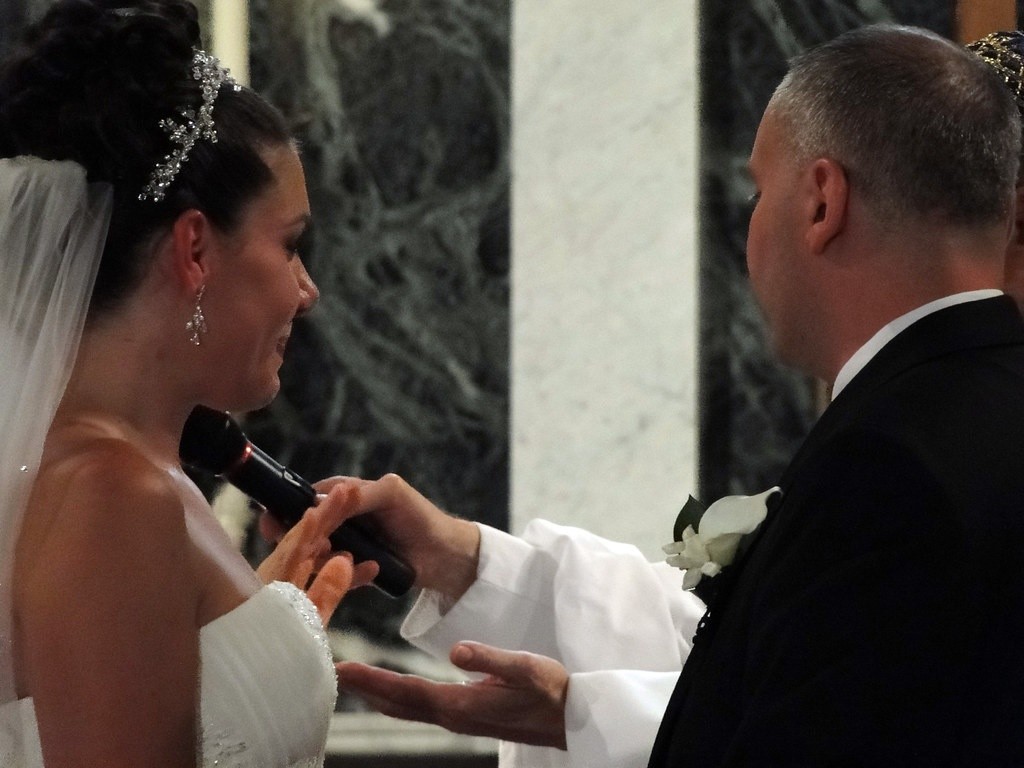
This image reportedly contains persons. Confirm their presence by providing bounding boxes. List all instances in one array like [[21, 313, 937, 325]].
[[0, 0, 379, 768], [260, 22, 1024, 768]]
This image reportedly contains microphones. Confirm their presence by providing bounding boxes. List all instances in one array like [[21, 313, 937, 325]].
[[178, 405, 416, 599]]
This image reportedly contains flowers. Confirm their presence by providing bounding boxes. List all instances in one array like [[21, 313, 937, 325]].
[[663, 485, 784, 590]]
